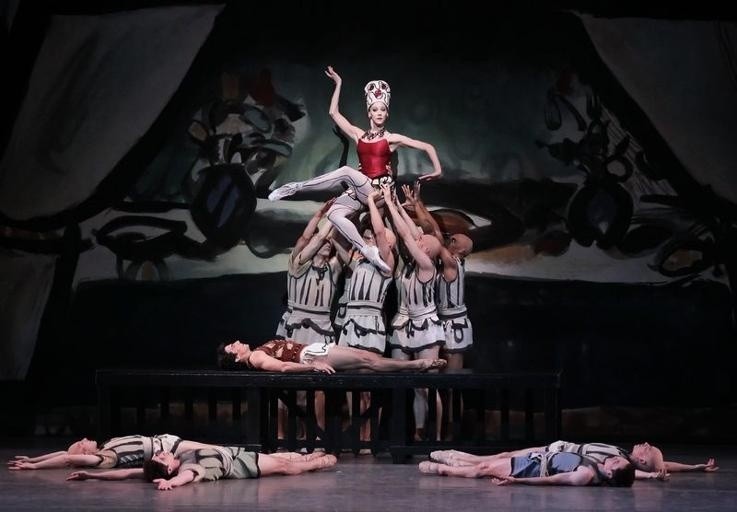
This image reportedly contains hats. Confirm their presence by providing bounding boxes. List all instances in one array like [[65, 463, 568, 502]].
[[363, 80, 391, 114]]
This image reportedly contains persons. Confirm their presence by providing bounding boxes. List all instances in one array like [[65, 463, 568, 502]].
[[269, 65, 441, 271], [215, 180, 474, 454], [67, 448, 337, 490], [6, 433, 261, 470], [418, 449, 636, 486], [431, 441, 719, 480]]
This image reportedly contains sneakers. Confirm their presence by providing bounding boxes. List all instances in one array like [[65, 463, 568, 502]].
[[313, 454, 338, 469], [430, 451, 453, 465], [268, 182, 297, 202], [369, 246, 394, 274], [416, 459, 444, 475], [420, 358, 448, 373], [307, 450, 325, 460]]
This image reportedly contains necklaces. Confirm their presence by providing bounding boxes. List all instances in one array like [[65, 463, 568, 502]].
[[361, 126, 387, 141]]
